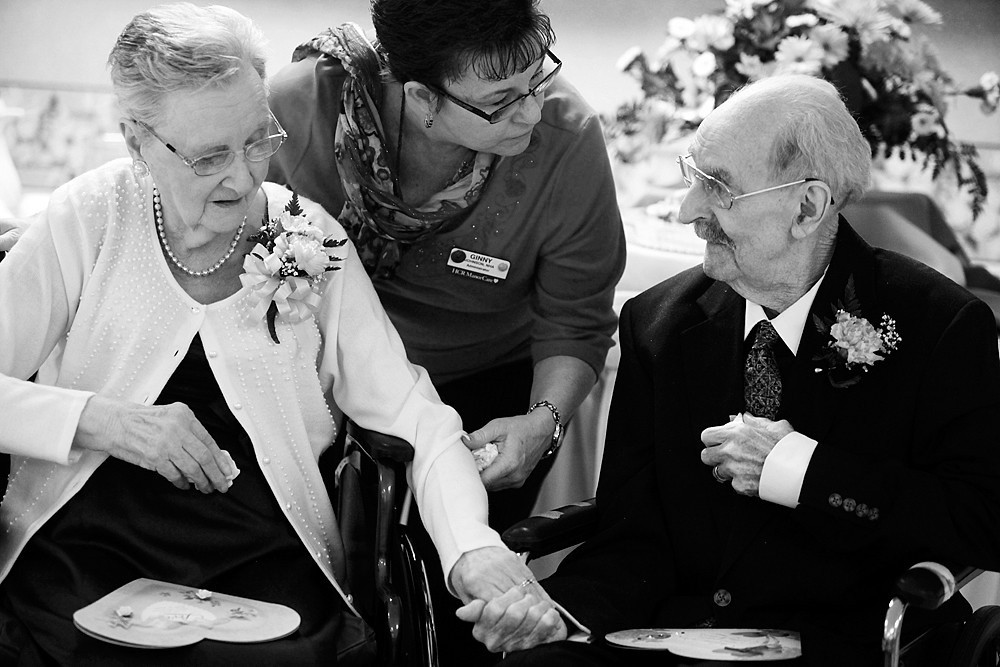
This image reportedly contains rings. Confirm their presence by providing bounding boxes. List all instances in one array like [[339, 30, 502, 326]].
[[713, 466, 727, 484], [521, 580, 531, 588]]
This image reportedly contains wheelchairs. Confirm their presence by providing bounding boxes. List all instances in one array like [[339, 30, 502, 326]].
[[0, 372, 440, 667], [500, 497, 1000, 667]]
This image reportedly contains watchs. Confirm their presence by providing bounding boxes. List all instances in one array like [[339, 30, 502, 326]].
[[527, 399, 565, 460]]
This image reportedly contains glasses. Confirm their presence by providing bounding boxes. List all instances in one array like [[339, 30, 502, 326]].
[[424, 48, 563, 125], [135, 109, 288, 176], [675, 154, 834, 210]]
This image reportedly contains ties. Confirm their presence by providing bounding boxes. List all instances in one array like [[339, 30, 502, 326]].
[[745, 322, 781, 420]]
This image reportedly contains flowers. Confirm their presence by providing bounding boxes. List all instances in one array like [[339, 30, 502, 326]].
[[596, 0, 1000, 221]]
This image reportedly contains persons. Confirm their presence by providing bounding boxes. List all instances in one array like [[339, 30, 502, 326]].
[[261, 0, 627, 667], [0, 3, 556, 667], [456, 72, 1000, 667]]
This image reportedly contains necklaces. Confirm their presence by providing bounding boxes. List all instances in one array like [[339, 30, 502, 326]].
[[393, 81, 476, 203], [149, 181, 248, 278]]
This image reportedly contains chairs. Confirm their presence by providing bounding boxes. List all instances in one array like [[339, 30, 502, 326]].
[[326, 417, 1000, 667]]
